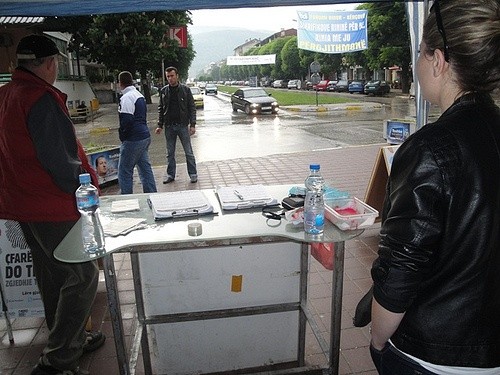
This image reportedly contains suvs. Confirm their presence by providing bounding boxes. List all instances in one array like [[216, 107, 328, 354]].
[[288, 80, 299, 89]]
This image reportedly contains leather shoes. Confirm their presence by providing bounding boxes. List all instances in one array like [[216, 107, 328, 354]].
[[31, 354, 90, 375], [79, 329, 106, 359]]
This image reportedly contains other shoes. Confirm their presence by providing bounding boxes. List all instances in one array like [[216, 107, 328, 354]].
[[191, 175, 197, 183], [163, 178, 174, 184]]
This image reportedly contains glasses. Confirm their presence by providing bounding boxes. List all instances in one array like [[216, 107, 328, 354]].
[[430, 0, 449, 63]]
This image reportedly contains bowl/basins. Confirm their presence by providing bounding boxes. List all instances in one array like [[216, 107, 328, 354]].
[[323, 198, 379, 231]]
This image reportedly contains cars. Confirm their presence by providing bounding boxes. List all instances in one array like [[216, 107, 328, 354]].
[[363, 80, 390, 94], [348, 81, 364, 94], [337, 80, 349, 92], [274, 80, 288, 88], [303, 80, 338, 91], [205, 84, 218, 95], [209, 80, 249, 86], [186, 82, 205, 94], [231, 88, 280, 115], [189, 87, 204, 109]]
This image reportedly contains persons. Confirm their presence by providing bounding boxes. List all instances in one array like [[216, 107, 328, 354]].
[[352, 0, 500, 375], [0, 34, 107, 375], [155, 66, 198, 184], [96, 156, 108, 185], [117, 71, 158, 194]]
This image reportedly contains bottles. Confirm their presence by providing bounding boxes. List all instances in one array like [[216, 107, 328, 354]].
[[304, 164, 325, 234], [76, 174, 106, 254]]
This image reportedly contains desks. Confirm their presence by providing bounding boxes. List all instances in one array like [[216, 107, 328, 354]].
[[54, 185, 365, 375]]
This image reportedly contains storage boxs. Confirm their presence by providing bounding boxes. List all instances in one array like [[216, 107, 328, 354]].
[[323, 197, 379, 230]]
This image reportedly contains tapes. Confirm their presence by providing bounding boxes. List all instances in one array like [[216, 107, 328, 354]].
[[188, 223, 202, 231]]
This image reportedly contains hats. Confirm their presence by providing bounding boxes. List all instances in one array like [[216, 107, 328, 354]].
[[16, 34, 69, 61]]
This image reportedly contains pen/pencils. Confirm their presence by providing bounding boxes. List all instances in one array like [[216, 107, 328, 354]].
[[233, 189, 243, 200], [147, 198, 152, 209]]
